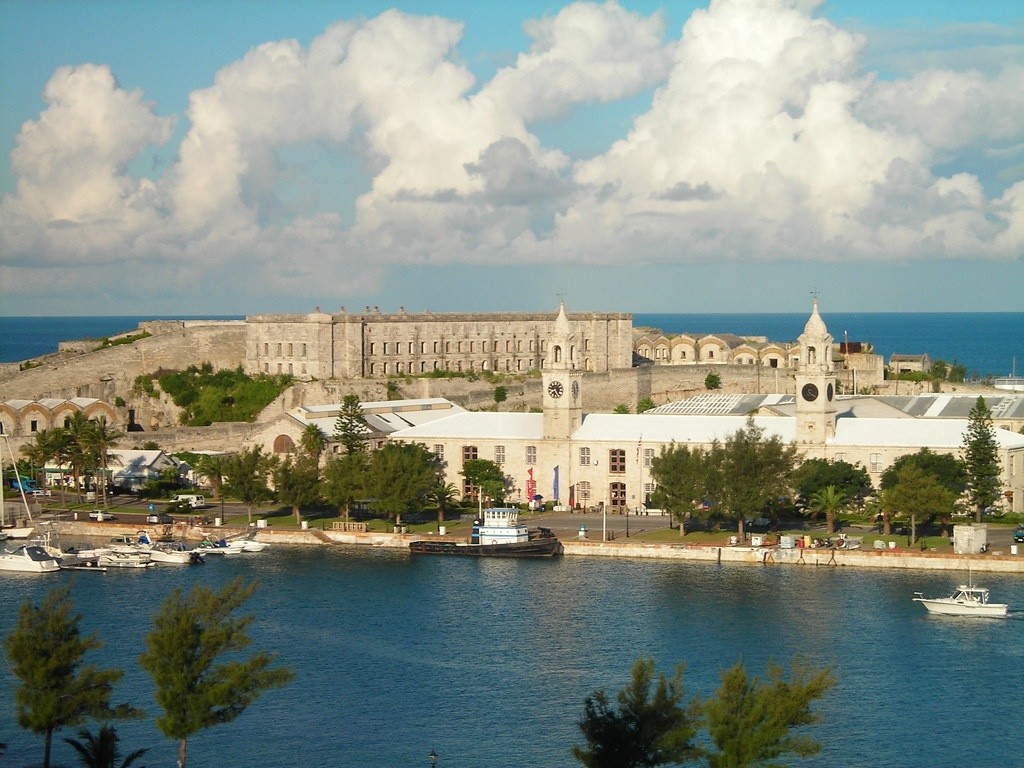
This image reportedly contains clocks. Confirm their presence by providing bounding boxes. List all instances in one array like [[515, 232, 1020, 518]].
[[802, 383, 819, 402], [570, 380, 580, 401], [548, 381, 564, 399]]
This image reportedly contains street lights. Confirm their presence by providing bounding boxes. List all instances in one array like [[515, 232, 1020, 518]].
[[501, 487, 509, 507]]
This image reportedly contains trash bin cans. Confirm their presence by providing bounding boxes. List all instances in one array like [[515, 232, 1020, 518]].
[[401, 525, 407, 534], [214, 518, 222, 527], [148, 503, 155, 512], [1010, 544, 1018, 555], [301, 521, 308, 530], [97, 513, 104, 522], [393, 524, 401, 533], [799, 539, 804, 548], [576, 503, 581, 510], [541, 504, 546, 512], [439, 526, 447, 535]]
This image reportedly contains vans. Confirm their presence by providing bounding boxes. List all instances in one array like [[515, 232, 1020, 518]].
[[348, 499, 387, 519], [170, 494, 206, 508]]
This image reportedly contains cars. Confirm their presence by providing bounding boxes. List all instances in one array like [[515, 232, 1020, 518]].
[[1013, 524, 1024, 542], [744, 518, 772, 528], [88, 509, 114, 520], [146, 513, 176, 524]]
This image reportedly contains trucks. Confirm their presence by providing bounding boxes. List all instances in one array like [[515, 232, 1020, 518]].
[[12, 476, 39, 494]]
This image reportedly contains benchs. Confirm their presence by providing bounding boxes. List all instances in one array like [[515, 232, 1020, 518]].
[[32, 490, 52, 497], [751, 533, 767, 541], [845, 536, 864, 544], [787, 532, 803, 541]]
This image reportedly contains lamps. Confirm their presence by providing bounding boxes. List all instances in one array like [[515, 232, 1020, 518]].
[[593, 460, 598, 466]]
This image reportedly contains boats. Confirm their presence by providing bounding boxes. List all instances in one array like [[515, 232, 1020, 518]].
[[911, 585, 1008, 617], [408, 507, 560, 558], [0, 421, 274, 573]]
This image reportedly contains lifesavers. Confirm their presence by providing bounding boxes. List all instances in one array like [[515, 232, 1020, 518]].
[[490, 539, 499, 546]]
